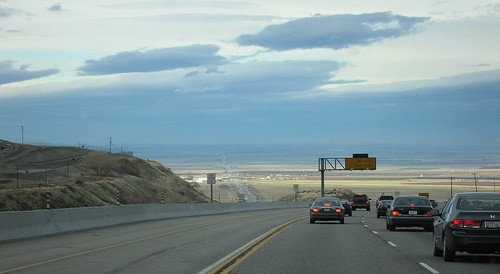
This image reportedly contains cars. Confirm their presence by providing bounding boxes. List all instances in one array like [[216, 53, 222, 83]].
[[376, 200, 393, 218], [430, 199, 437, 209], [433, 192, 500, 263], [310, 197, 345, 224], [340, 199, 353, 216], [385, 196, 436, 232]]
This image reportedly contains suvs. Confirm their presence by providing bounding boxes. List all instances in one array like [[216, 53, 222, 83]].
[[351, 193, 372, 211], [376, 195, 394, 208]]
